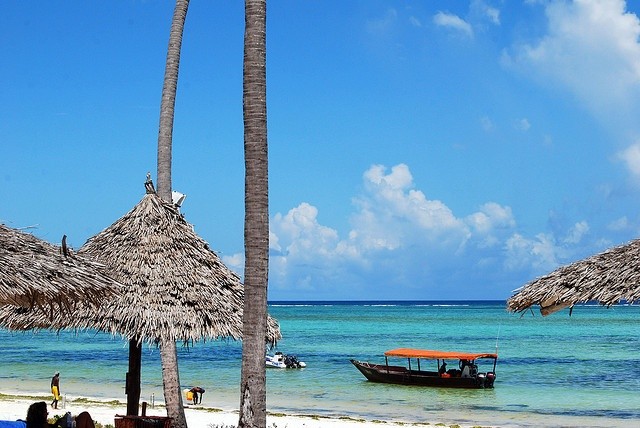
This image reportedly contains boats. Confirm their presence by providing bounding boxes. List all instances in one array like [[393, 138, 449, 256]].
[[265, 348, 307, 367], [350, 319, 501, 388]]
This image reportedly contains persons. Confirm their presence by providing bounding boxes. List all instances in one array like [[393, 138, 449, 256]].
[[50, 371, 60, 409], [26, 402, 95, 428], [439, 359, 477, 378], [189, 387, 205, 405]]
